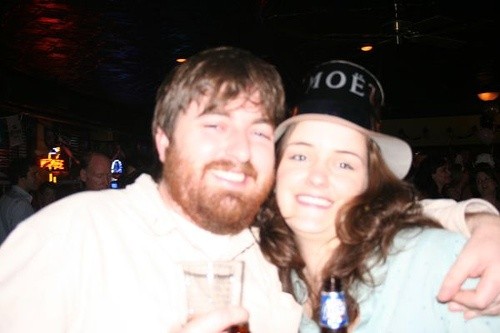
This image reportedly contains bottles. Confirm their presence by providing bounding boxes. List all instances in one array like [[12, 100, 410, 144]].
[[318, 277, 349, 333]]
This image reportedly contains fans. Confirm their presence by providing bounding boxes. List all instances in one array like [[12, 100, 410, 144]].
[[324, 1, 468, 54]]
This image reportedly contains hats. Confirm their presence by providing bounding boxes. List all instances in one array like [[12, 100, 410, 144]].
[[272, 60, 414, 182]]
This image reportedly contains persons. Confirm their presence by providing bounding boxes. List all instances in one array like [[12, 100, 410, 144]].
[[470, 162, 500, 213], [448, 165, 476, 202], [38, 181, 58, 208], [414, 155, 455, 201], [0, 46, 500, 333], [79, 150, 112, 191], [248, 59, 500, 333], [0, 156, 41, 245]]
